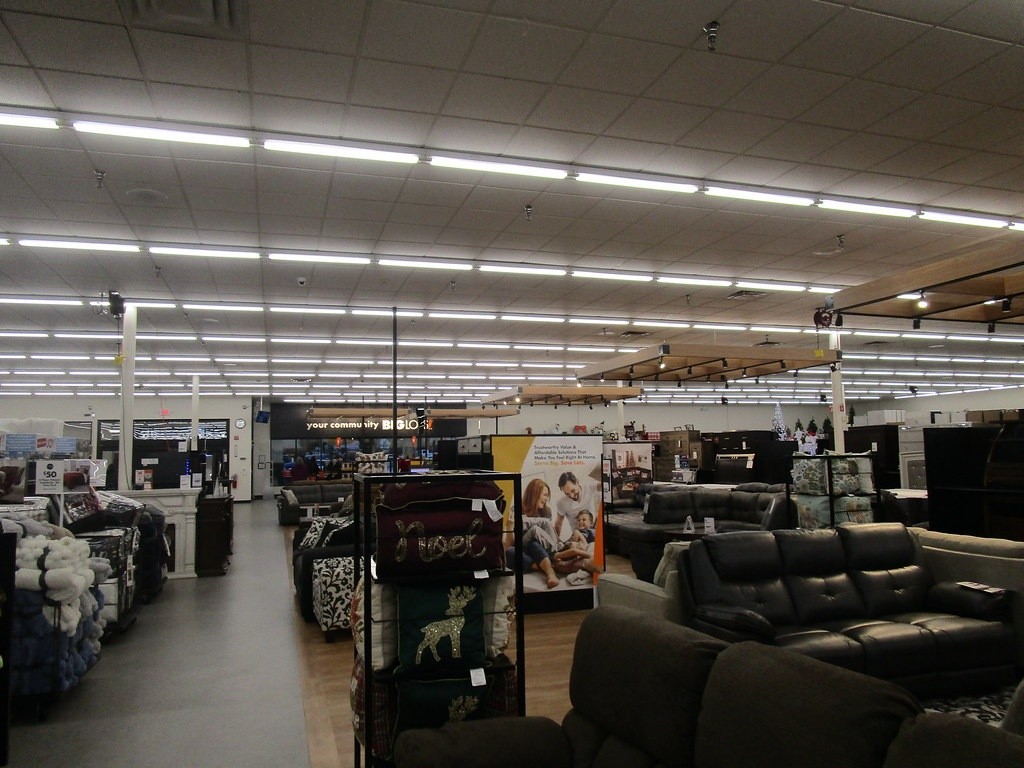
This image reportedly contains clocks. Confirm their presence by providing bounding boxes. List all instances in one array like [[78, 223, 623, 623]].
[[236, 419, 246, 429]]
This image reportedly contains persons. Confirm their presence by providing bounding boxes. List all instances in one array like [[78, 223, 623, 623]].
[[290, 456, 319, 480], [505, 472, 602, 589], [401, 453, 411, 472]]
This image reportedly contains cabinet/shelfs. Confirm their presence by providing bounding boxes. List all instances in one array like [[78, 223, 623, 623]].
[[652, 430, 714, 481], [106, 487, 234, 580], [353, 469, 525, 768]]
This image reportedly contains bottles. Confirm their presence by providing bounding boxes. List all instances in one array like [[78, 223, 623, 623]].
[[219, 483, 223, 496]]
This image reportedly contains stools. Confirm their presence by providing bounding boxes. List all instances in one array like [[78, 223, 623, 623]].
[[312, 555, 374, 643]]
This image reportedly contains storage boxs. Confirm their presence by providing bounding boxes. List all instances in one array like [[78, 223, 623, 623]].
[[853, 408, 905, 427], [934, 409, 1024, 424]]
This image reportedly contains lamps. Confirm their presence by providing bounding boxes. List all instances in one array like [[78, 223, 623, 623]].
[[482, 311, 844, 410], [412, 431, 416, 447], [913, 290, 1010, 333], [336, 432, 341, 449]]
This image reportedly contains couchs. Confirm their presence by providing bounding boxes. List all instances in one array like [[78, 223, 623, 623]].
[[277, 478, 376, 624], [604, 482, 787, 558], [597, 522, 1024, 768]]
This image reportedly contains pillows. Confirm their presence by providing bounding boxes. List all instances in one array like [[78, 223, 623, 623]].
[[348, 471, 519, 757], [299, 517, 355, 548], [789, 449, 878, 530], [281, 489, 299, 505], [355, 451, 388, 473]]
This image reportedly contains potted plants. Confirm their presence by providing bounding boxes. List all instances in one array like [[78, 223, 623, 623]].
[[787, 416, 834, 441]]
